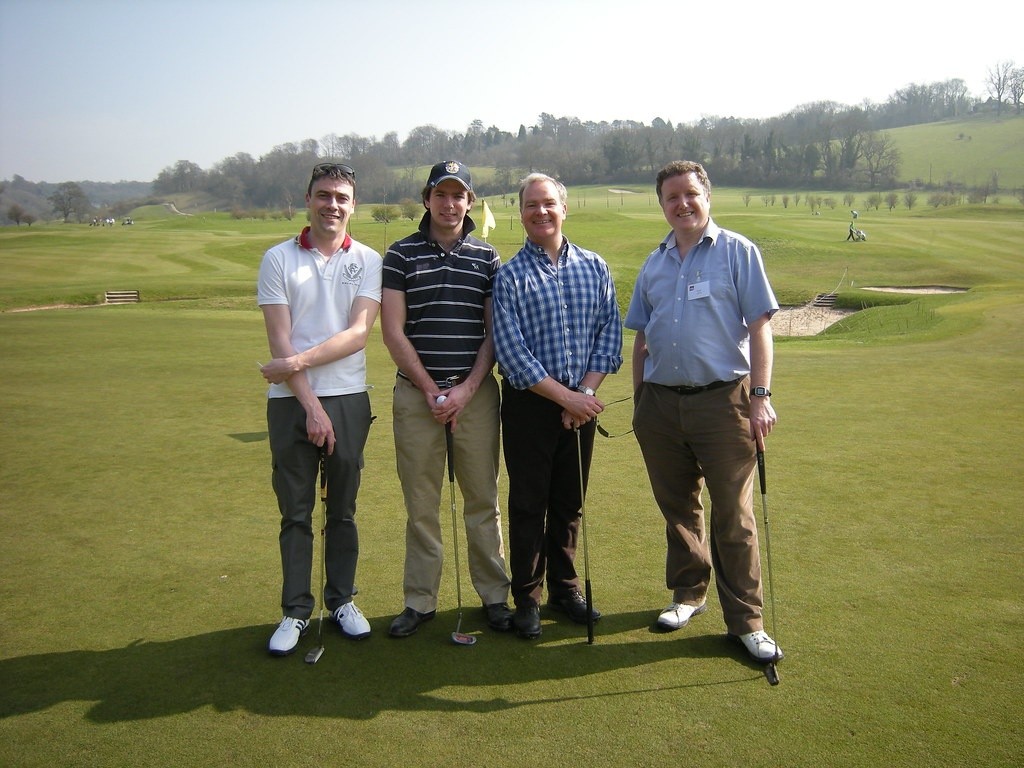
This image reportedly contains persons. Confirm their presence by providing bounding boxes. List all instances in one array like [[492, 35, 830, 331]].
[[624, 161, 788, 662], [382, 159, 515, 638], [847, 221, 855, 241], [492, 172, 624, 640], [256, 160, 383, 655], [851, 210, 858, 218], [815, 212, 820, 215]]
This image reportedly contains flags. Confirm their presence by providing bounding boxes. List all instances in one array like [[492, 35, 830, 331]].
[[481, 201, 496, 239]]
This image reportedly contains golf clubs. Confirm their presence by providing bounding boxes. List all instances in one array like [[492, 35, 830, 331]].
[[304, 438, 328, 664], [570, 419, 594, 644], [755, 436, 779, 686], [444, 421, 477, 645]]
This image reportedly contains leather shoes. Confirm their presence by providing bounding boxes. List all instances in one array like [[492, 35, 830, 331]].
[[513, 604, 544, 639], [546, 593, 600, 623], [480, 602, 515, 631], [388, 607, 435, 637]]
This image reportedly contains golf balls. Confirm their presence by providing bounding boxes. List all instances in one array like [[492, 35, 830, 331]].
[[437, 395, 447, 405]]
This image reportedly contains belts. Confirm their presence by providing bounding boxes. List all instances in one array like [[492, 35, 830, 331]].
[[398, 371, 471, 391], [659, 379, 738, 396]]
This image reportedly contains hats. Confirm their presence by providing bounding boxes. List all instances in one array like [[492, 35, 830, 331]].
[[425, 160, 471, 192]]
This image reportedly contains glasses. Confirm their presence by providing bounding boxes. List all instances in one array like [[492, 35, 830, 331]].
[[314, 163, 355, 180], [592, 396, 638, 439]]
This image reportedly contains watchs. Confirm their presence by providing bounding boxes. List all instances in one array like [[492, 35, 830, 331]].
[[577, 385, 595, 397], [750, 386, 771, 397]]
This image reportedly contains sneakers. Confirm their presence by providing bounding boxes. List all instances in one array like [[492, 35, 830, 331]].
[[326, 602, 371, 639], [657, 597, 708, 628], [728, 630, 783, 663], [268, 617, 312, 657]]
